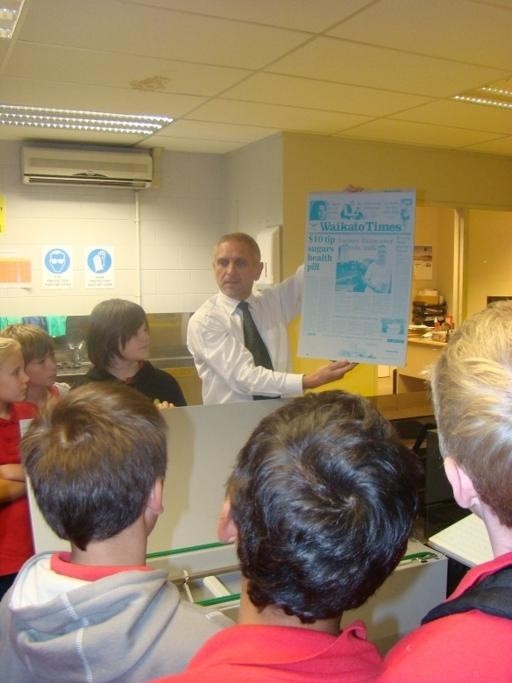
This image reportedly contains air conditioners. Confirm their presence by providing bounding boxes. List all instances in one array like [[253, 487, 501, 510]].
[[21, 146, 153, 190]]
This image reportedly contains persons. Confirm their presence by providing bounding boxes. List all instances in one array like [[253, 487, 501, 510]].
[[145, 387, 422, 682], [375, 297, 511, 682], [341, 198, 360, 220], [184, 181, 367, 404], [0, 380, 239, 681], [84, 297, 188, 406], [382, 318, 404, 334], [0, 322, 72, 418], [309, 198, 326, 218], [0, 335, 41, 598], [355, 241, 391, 294]]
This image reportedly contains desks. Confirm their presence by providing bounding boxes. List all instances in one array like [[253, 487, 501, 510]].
[[364, 391, 497, 599]]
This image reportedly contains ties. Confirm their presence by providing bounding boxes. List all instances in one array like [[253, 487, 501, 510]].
[[236, 300, 279, 400]]
